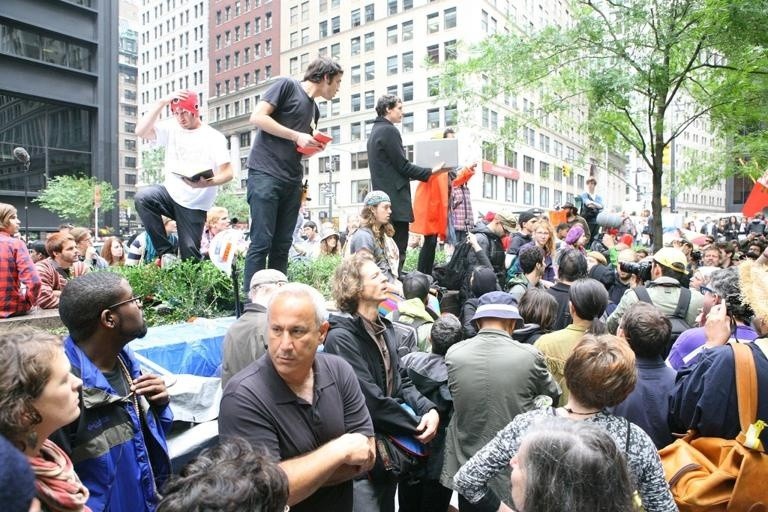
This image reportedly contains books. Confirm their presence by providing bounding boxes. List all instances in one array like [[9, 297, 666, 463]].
[[296, 131, 333, 157]]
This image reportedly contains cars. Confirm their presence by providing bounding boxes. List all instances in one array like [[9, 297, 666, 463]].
[[696, 213, 748, 244]]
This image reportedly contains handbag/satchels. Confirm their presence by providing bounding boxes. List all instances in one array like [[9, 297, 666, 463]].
[[657, 430, 767, 512], [369, 395, 429, 489]]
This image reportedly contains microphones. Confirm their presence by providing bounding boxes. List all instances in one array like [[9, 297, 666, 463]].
[[13, 146, 31, 166]]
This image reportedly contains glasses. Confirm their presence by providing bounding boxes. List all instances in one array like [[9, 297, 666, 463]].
[[106, 295, 143, 310], [700, 284, 715, 296]]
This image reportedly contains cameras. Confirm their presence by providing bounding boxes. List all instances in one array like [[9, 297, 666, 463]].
[[690, 251, 701, 260], [619, 260, 652, 281], [725, 293, 753, 326], [755, 233, 762, 237]]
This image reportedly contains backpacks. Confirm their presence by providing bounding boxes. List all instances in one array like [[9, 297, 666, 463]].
[[634, 284, 692, 348], [391, 308, 432, 358]]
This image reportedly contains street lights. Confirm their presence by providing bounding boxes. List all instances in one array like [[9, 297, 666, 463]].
[[320, 169, 336, 223], [14, 147, 30, 245], [126, 206, 131, 232]]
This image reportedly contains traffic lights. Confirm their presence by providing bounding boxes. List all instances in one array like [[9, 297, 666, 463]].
[[662, 144, 669, 165], [561, 164, 570, 178], [661, 195, 667, 207]]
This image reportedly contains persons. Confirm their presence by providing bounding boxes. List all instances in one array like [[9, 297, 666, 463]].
[[244, 55, 343, 304], [367, 94, 452, 280], [442, 130, 474, 246], [1, 178, 767, 511], [134, 90, 233, 270]]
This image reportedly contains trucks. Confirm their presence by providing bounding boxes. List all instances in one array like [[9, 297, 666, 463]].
[[660, 213, 688, 232]]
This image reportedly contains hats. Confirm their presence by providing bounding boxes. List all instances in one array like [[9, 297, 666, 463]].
[[171, 91, 199, 117], [496, 210, 518, 235], [519, 211, 532, 226], [470, 292, 524, 331], [655, 247, 689, 275]]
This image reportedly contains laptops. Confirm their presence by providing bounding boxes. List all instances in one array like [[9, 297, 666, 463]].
[[411, 137, 460, 170]]
